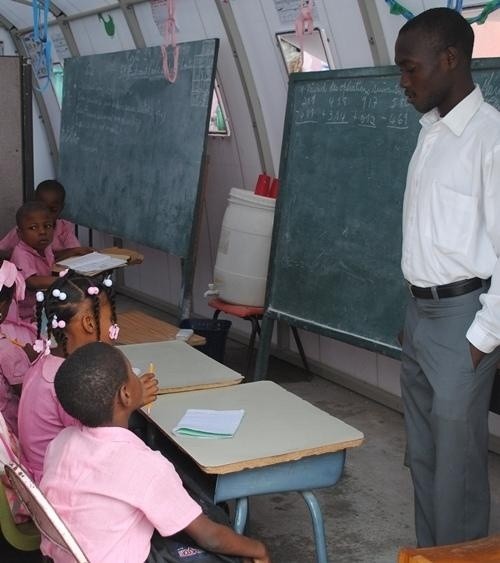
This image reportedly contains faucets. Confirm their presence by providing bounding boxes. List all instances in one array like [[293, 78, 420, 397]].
[[201, 284, 219, 298]]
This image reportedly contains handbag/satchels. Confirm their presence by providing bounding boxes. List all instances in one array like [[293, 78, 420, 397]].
[[146, 447, 241, 563]]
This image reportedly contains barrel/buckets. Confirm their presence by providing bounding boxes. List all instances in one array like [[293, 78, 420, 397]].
[[211, 188, 277, 308]]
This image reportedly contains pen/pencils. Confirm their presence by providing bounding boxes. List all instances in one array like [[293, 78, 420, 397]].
[[11, 340, 24, 348], [147, 362, 153, 415]]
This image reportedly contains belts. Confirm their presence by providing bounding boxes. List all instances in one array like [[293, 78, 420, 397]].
[[412, 277, 491, 298]]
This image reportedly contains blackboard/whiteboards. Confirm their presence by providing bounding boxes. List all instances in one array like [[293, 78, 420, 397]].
[[57, 38, 220, 260], [263, 57, 500, 360]]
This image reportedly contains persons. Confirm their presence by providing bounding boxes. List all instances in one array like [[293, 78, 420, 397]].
[[10, 200, 96, 324], [0, 179, 81, 259], [39, 340, 271, 563], [392, 6, 500, 547], [0, 258, 46, 441], [17, 267, 158, 485]]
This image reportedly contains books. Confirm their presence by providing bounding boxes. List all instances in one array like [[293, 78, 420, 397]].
[[172, 408, 245, 439], [55, 250, 128, 277]]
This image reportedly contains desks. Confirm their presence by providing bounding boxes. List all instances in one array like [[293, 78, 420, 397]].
[[116, 309, 205, 346], [137, 379, 366, 561], [113, 338, 245, 394]]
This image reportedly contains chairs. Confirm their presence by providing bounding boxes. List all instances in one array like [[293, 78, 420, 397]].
[[0, 414, 88, 563], [209, 298, 313, 382]]
